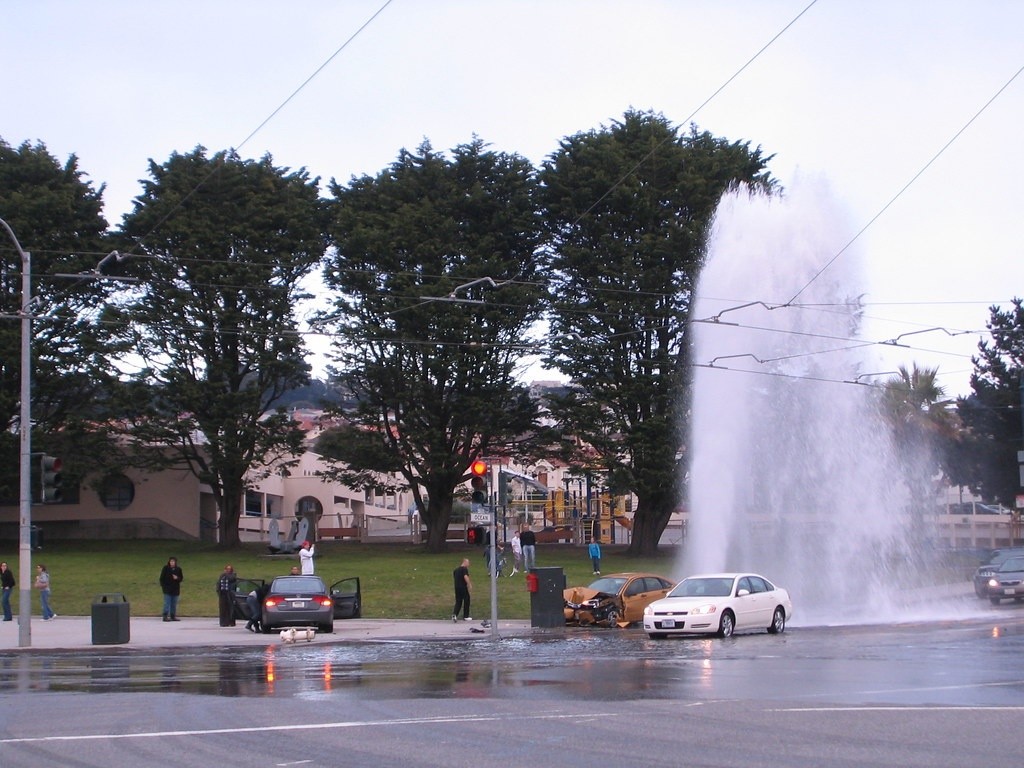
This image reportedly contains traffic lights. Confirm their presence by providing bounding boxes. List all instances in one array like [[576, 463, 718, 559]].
[[471, 459, 488, 504], [466, 526, 486, 545], [42, 455, 63, 504], [498, 472, 514, 507]]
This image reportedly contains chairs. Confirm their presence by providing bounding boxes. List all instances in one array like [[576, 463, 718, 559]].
[[278, 581, 289, 591]]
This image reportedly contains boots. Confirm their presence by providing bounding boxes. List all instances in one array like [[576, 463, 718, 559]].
[[254, 623, 262, 633], [245, 622, 254, 632]]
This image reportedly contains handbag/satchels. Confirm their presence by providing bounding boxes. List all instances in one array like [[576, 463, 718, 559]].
[[34, 581, 48, 589]]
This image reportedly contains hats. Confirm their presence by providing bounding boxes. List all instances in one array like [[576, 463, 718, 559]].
[[302, 541, 310, 548]]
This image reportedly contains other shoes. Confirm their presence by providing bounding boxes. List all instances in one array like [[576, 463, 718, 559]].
[[162, 613, 171, 621], [593, 571, 600, 576], [40, 618, 51, 622], [51, 614, 57, 619], [513, 567, 519, 573], [452, 614, 457, 623], [464, 617, 472, 621], [3, 617, 12, 621], [169, 615, 181, 621]]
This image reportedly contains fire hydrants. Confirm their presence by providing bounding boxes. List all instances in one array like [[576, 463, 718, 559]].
[[280, 628, 314, 644]]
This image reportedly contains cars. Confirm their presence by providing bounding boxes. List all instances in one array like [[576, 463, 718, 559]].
[[533, 524, 573, 544], [642, 572, 793, 640], [563, 573, 677, 629], [988, 556, 1024, 604], [973, 547, 1024, 598], [228, 575, 362, 633]]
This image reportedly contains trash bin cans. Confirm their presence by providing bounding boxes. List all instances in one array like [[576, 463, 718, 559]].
[[91, 593, 131, 645]]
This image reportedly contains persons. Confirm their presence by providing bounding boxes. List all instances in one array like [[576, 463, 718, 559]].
[[483, 542, 505, 576], [0, 562, 16, 621], [451, 558, 472, 624], [520, 523, 535, 573], [34, 564, 57, 622], [296, 541, 316, 576], [588, 537, 601, 575], [245, 584, 271, 633], [511, 531, 522, 573], [290, 566, 300, 575], [159, 557, 183, 622], [216, 565, 237, 627]]
